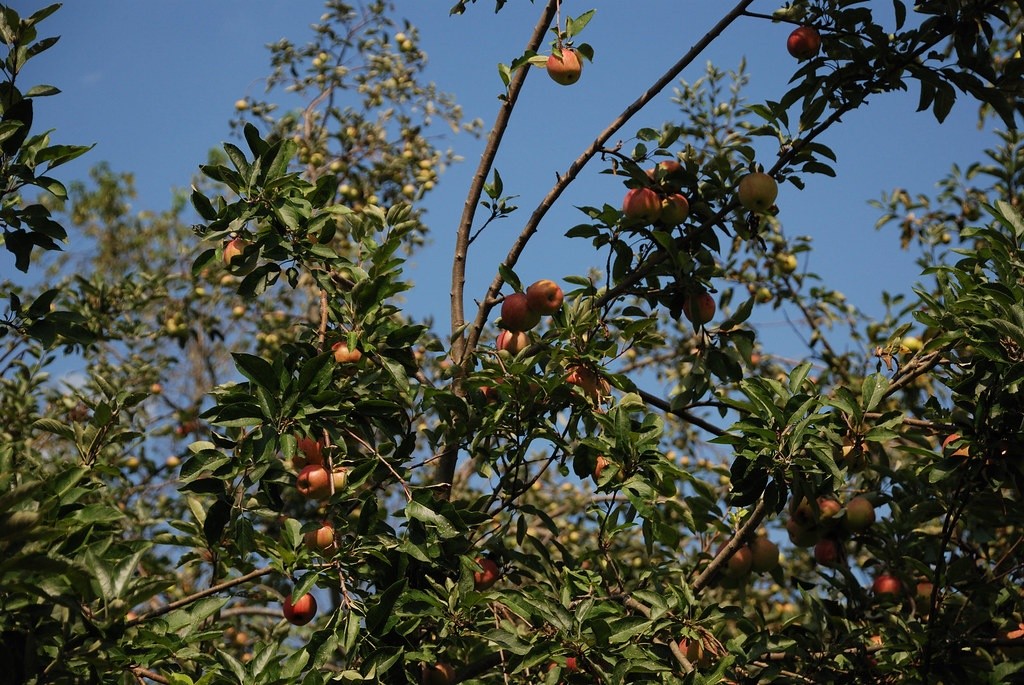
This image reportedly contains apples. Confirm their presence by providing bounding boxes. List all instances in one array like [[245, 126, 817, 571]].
[[100, 24, 1024, 685]]
